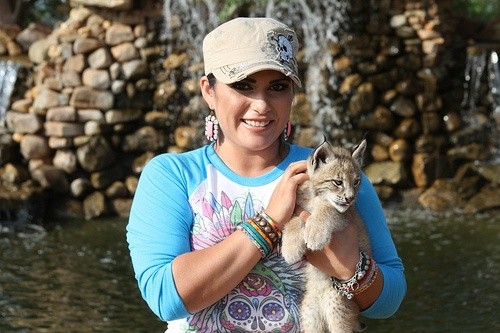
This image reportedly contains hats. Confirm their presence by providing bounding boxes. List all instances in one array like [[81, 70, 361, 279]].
[[202, 17, 302, 89]]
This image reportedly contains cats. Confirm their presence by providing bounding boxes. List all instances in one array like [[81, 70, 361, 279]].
[[278, 132, 367, 333]]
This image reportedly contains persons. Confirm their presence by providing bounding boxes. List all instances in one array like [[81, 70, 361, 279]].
[[125, 17, 407, 333]]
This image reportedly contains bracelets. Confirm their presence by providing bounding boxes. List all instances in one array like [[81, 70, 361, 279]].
[[236, 210, 283, 258], [331, 250, 380, 299]]
